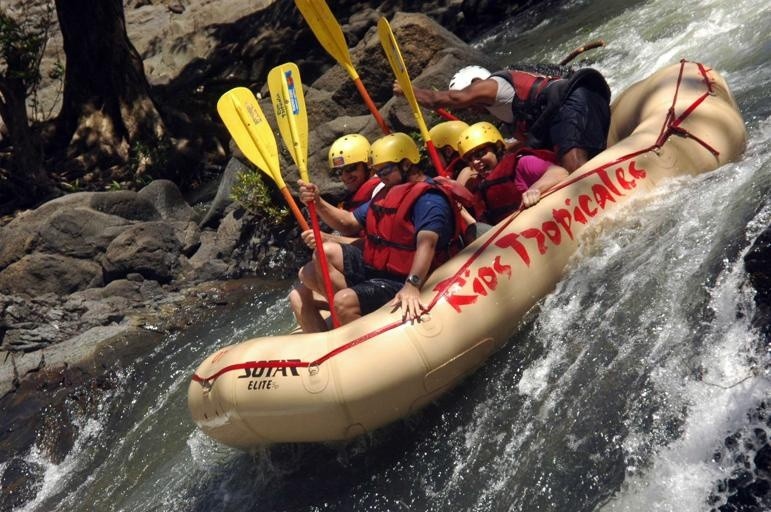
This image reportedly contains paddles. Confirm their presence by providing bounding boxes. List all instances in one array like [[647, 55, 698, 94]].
[[217, 86, 311, 233], [377, 17, 468, 235], [268, 62, 342, 330], [295, 0, 392, 136]]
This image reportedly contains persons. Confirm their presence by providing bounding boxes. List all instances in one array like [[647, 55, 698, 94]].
[[289, 65, 611, 333]]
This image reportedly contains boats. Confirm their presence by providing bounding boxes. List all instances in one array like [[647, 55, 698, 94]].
[[187, 59, 746, 448]]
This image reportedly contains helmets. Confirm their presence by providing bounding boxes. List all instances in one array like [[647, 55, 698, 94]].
[[327, 133, 372, 170], [424, 120, 470, 153], [458, 121, 506, 159], [370, 131, 421, 167], [449, 64, 491, 93]]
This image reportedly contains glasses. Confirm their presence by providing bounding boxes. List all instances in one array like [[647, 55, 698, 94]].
[[332, 164, 358, 178], [376, 166, 395, 178], [465, 149, 488, 162]]
